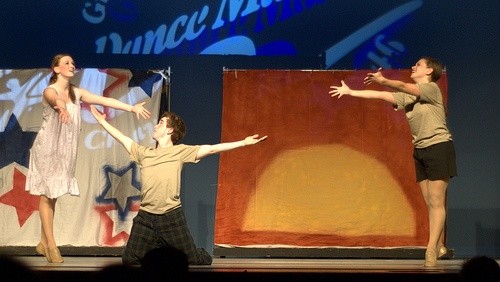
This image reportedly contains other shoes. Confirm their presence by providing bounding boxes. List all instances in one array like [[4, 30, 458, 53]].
[[424, 250, 437, 267], [437, 247, 447, 258], [36, 242, 63, 263]]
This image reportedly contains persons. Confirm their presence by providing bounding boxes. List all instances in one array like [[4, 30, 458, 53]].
[[459, 256, 500, 282], [26, 54, 151, 264], [0, 255, 36, 282], [99, 246, 189, 282], [91, 105, 267, 264], [329, 57, 456, 266]]
